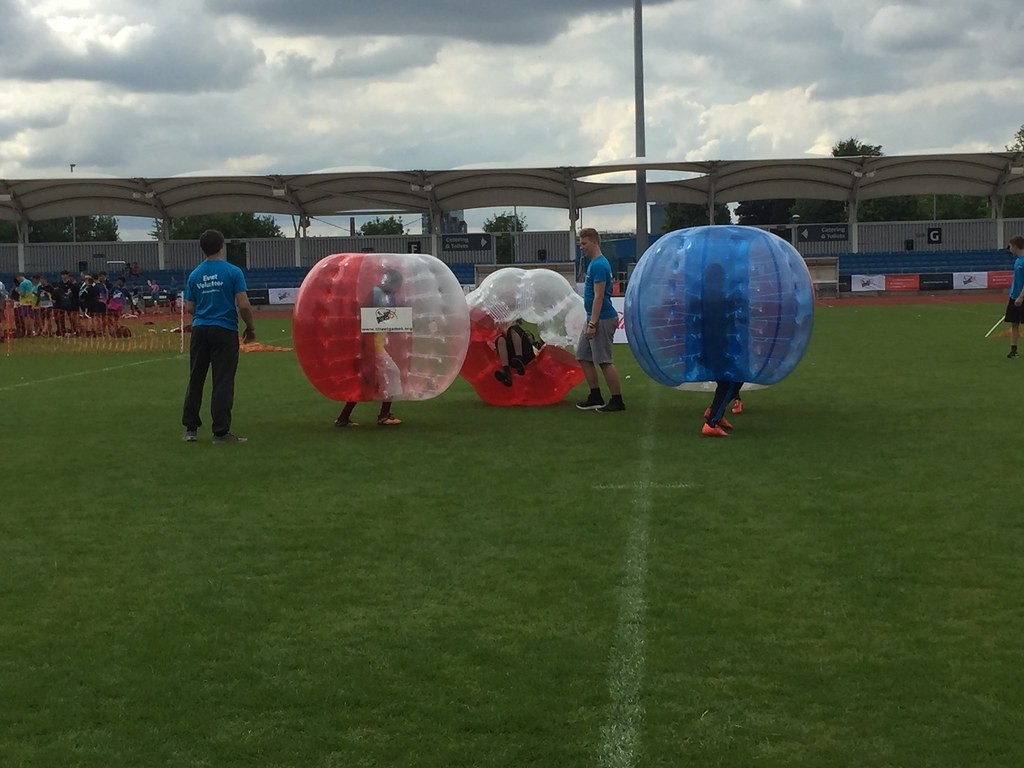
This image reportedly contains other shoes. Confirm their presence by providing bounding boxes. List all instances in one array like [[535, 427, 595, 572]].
[[1006, 352, 1020, 359], [510, 358, 525, 376], [0, 306, 179, 343], [493, 370, 512, 387]]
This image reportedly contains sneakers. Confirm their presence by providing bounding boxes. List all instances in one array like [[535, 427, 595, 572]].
[[214, 433, 248, 444], [576, 395, 606, 410], [596, 398, 626, 413], [701, 423, 729, 437], [377, 415, 402, 425], [182, 429, 198, 441], [333, 420, 359, 428], [731, 399, 744, 415], [703, 407, 733, 429]]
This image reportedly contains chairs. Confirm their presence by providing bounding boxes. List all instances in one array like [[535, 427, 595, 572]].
[[617, 248, 1016, 291], [0, 259, 575, 302]]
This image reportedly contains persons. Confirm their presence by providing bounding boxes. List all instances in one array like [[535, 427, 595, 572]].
[[334, 268, 402, 427], [696, 262, 745, 436], [183, 228, 256, 444], [575, 228, 619, 412], [0, 262, 145, 340], [149, 279, 161, 315], [169, 275, 180, 315], [492, 320, 542, 387], [1006, 237, 1024, 359]]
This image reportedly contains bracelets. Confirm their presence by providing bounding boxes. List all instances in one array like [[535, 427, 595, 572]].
[[246, 328, 255, 331], [588, 321, 596, 329]]
